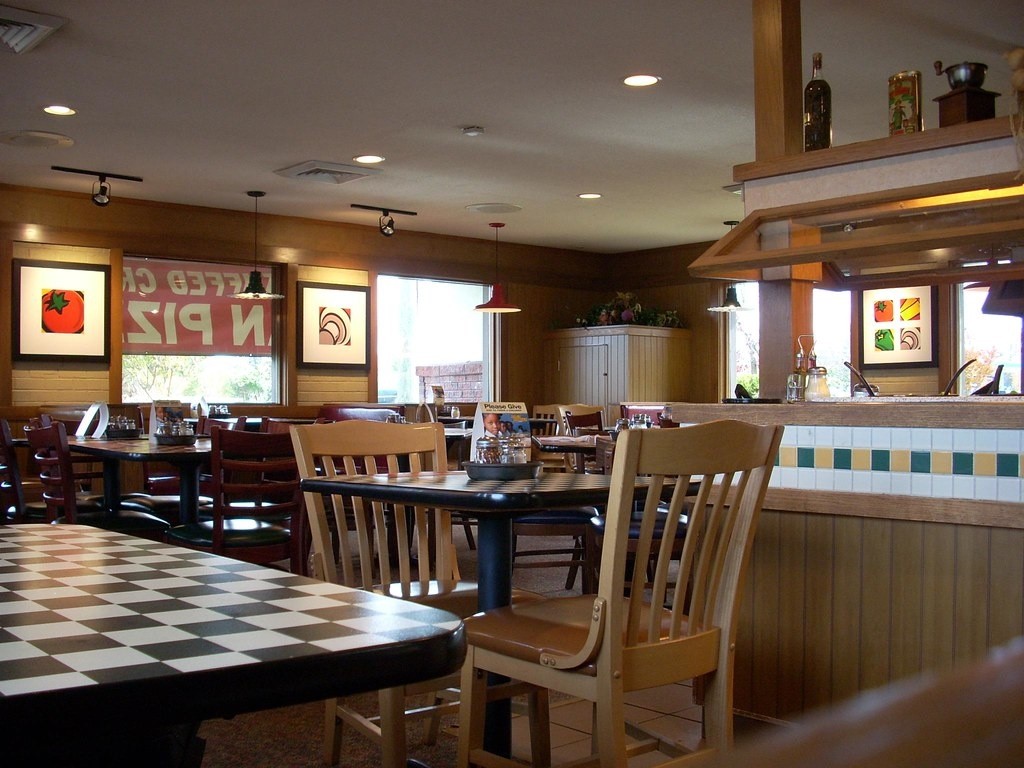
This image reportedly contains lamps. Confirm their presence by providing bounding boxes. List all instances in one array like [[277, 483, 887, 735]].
[[226, 191, 286, 301], [474, 223, 522, 313], [349, 204, 418, 237], [50, 165, 143, 207], [706, 220, 744, 312]]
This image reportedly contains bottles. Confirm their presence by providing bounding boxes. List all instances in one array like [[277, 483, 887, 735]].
[[192, 407, 197, 417], [444, 405, 460, 418], [197, 403, 202, 416], [107, 416, 136, 431], [792, 353, 804, 374], [473, 436, 526, 465], [613, 415, 653, 431], [389, 414, 406, 424], [208, 405, 230, 415], [159, 418, 193, 437], [804, 51, 833, 152], [663, 403, 673, 420], [805, 367, 829, 399]]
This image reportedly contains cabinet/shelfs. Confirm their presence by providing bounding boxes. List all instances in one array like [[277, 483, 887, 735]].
[[545, 325, 693, 428]]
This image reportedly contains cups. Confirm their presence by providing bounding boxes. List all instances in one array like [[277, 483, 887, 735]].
[[787, 374, 803, 401]]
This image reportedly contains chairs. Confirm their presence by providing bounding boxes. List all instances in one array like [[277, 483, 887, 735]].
[[0, 404, 787, 768]]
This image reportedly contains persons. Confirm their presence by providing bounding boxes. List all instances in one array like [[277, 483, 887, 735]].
[[155, 407, 184, 428], [482, 412, 526, 441]]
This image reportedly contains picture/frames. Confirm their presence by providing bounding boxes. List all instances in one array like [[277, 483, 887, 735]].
[[12, 258, 111, 363], [859, 285, 941, 370], [296, 281, 370, 370]]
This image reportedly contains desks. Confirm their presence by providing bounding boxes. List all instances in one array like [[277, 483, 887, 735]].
[[11, 433, 212, 524], [438, 416, 557, 429], [301, 471, 704, 768], [0, 524, 469, 768], [444, 427, 473, 471], [183, 418, 334, 426]]
